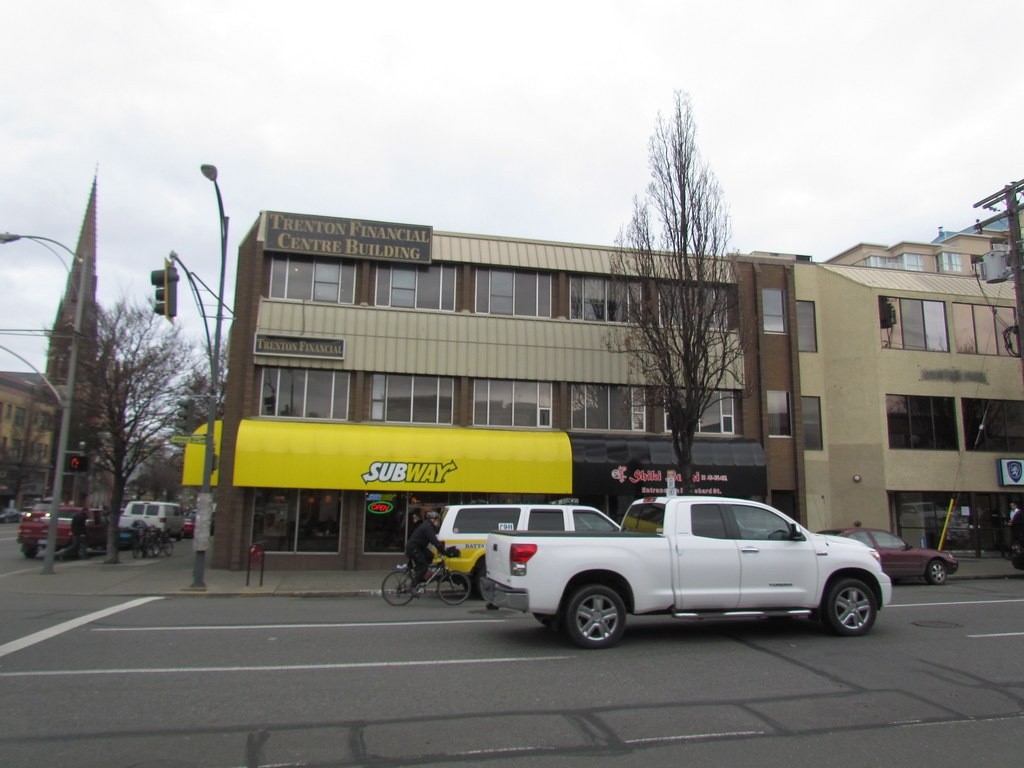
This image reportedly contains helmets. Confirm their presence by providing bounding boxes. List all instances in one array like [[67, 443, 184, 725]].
[[426, 512, 441, 519]]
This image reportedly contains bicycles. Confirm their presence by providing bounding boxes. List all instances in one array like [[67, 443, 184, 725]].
[[132, 520, 174, 557], [381, 554, 472, 607]]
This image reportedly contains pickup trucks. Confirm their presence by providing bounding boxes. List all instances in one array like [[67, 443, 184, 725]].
[[480, 496, 892, 649], [902, 502, 947, 520]]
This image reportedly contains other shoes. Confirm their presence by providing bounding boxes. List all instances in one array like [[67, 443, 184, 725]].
[[407, 586, 418, 597]]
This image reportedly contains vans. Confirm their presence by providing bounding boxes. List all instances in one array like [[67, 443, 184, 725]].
[[433, 504, 620, 601], [123, 501, 184, 542]]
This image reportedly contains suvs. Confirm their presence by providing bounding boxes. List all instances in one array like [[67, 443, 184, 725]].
[[17, 506, 109, 559]]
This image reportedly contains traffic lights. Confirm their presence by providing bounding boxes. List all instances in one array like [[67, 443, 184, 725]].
[[152, 258, 179, 324], [68, 453, 88, 470], [176, 398, 195, 437]]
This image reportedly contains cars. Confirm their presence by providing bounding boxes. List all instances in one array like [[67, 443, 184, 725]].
[[183, 509, 204, 539], [0, 508, 20, 523], [814, 528, 958, 585], [117, 517, 150, 550]]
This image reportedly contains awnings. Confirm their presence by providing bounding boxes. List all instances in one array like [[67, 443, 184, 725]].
[[566, 432, 767, 496], [183, 419, 573, 496]]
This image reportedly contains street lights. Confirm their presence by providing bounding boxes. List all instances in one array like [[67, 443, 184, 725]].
[[189, 163, 230, 588], [0, 230, 90, 575]]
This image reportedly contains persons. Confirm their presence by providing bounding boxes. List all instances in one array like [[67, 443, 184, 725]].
[[407, 513, 423, 574], [100, 506, 108, 523], [71, 507, 89, 559], [1006, 500, 1023, 560], [406, 512, 448, 590]]
